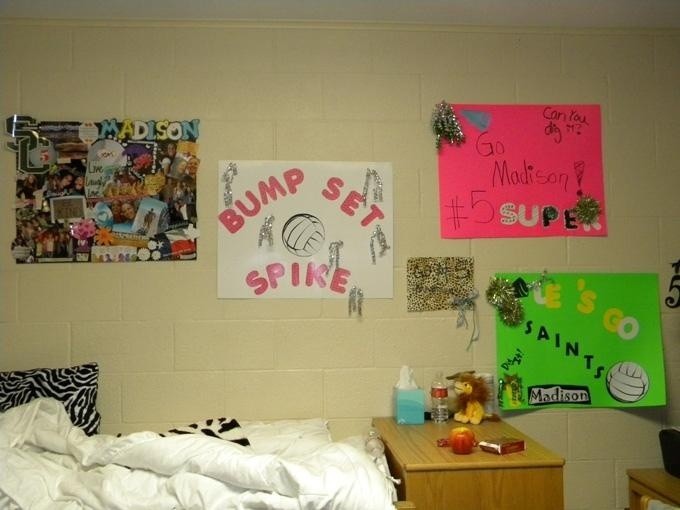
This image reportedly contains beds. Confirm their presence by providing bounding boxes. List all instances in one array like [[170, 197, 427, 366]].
[[0, 398, 416, 509]]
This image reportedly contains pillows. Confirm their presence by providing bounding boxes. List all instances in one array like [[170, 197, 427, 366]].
[[154, 412, 256, 451], [240, 414, 337, 454], [0, 357, 106, 440]]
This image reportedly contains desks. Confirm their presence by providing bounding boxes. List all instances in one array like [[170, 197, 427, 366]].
[[618, 464, 680, 509]]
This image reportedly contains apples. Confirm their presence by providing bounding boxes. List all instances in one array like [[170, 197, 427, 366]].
[[450, 427, 474, 454]]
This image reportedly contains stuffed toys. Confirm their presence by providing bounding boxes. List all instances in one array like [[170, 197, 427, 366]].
[[454, 373, 499, 423]]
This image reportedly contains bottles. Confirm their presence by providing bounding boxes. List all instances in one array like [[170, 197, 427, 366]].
[[431, 369, 449, 425], [478, 374, 495, 418], [364, 427, 385, 458]]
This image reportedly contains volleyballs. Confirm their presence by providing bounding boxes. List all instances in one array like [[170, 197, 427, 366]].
[[606, 362, 649, 403], [282, 214, 325, 256]]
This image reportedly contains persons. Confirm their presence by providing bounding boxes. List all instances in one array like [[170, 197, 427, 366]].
[[15, 142, 200, 262]]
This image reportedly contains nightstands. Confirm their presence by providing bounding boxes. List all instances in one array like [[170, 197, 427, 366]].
[[370, 409, 569, 510]]
